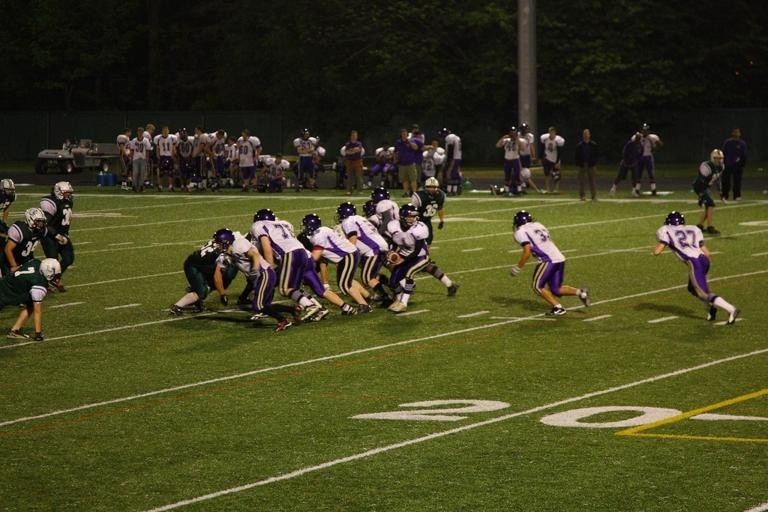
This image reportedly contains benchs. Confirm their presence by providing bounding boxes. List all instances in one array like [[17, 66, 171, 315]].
[[323, 163, 369, 174]]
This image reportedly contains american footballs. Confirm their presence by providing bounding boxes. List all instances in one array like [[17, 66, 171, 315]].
[[386, 251, 401, 264]]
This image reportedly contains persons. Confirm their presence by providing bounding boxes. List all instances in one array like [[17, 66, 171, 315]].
[[694, 149, 725, 236], [171, 196, 460, 331], [609, 132, 643, 199], [538, 127, 566, 194], [631, 121, 665, 196], [1, 177, 75, 341], [117, 124, 463, 196], [490, 123, 540, 196], [508, 208, 590, 316], [575, 128, 599, 199], [654, 210, 741, 326], [720, 127, 749, 201]]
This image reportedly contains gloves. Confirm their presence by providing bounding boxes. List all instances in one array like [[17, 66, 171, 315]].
[[34, 332, 43, 341], [511, 266, 521, 276]]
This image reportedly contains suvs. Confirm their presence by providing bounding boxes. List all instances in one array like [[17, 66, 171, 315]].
[[35, 136, 112, 174]]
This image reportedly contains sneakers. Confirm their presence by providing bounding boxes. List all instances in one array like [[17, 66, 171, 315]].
[[706, 306, 717, 321], [697, 224, 707, 232], [579, 288, 590, 307], [727, 308, 740, 324], [707, 226, 721, 234], [545, 305, 567, 316], [6, 328, 29, 339], [169, 282, 460, 332]]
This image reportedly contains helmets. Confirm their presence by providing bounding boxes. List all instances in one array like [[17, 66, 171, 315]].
[[213, 202, 420, 253], [0, 178, 74, 289], [664, 211, 684, 225], [513, 209, 532, 232]]
